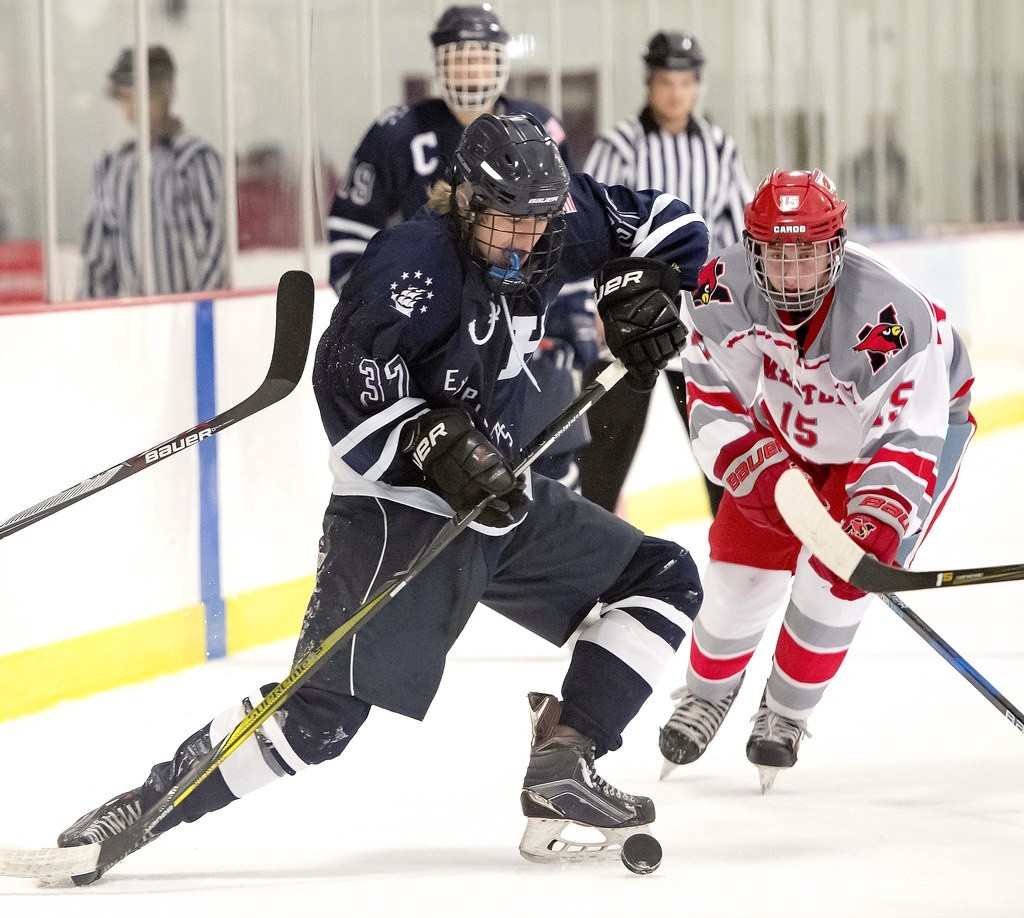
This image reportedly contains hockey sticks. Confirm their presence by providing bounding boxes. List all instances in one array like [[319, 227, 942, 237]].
[[0, 266, 317, 542], [874, 588, 1024, 735], [0, 356, 632, 882], [773, 463, 1023, 595]]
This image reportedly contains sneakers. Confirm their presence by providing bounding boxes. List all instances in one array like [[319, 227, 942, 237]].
[[39, 761, 178, 890], [658, 668, 746, 780], [519, 691, 656, 863], [745, 677, 815, 796]]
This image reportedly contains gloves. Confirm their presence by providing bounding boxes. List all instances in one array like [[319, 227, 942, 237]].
[[712, 433, 831, 537], [408, 404, 529, 528], [807, 493, 912, 601], [592, 255, 689, 394]]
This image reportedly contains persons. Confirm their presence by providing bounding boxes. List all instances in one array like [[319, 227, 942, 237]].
[[76, 45, 236, 292], [327, 6, 616, 498], [33, 108, 704, 885], [578, 30, 758, 519], [654, 165, 978, 794]]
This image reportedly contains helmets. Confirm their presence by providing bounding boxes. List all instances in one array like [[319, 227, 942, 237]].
[[429, 5, 512, 112], [643, 31, 703, 86], [445, 110, 571, 299], [740, 166, 847, 330], [106, 42, 173, 93]]
